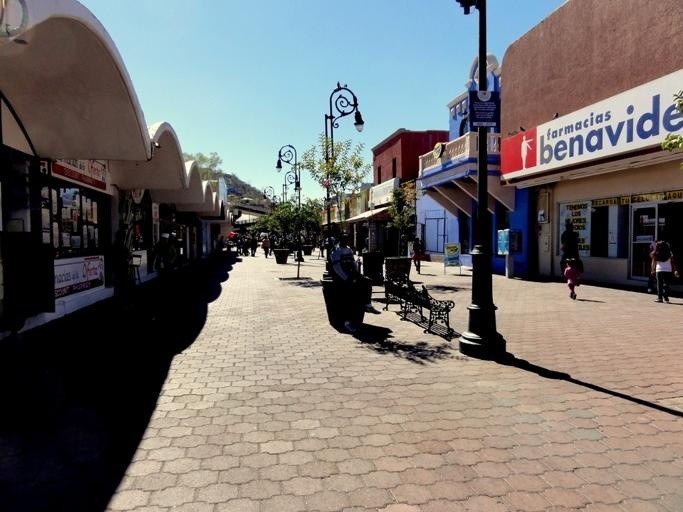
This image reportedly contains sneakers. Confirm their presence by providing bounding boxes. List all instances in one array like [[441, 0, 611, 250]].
[[366, 306, 381, 314], [570, 292, 576, 300], [654, 299, 669, 303], [345, 324, 356, 332]]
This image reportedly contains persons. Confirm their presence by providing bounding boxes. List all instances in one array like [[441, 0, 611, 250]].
[[330, 234, 382, 333], [216, 235, 224, 250], [228, 229, 326, 258], [110, 230, 133, 310], [413, 238, 423, 274], [560, 218, 578, 276], [650, 241, 678, 304], [565, 257, 579, 300]]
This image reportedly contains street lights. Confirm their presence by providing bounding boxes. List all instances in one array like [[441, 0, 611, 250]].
[[275, 144, 304, 262], [264, 186, 281, 211], [323, 81, 364, 278]]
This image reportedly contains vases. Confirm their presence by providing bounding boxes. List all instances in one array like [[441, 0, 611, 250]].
[[303, 244, 312, 255], [320, 277, 371, 333], [274, 249, 289, 264]]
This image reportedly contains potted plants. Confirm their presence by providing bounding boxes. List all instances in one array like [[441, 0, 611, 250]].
[[384, 182, 418, 280]]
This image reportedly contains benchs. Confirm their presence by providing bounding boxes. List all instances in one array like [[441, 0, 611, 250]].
[[383, 279, 454, 335]]
